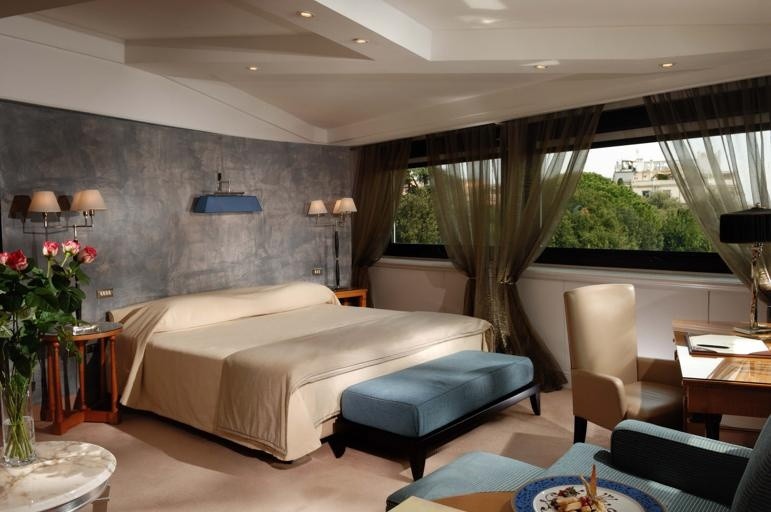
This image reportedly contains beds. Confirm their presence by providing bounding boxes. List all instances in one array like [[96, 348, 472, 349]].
[[105, 279, 496, 465]]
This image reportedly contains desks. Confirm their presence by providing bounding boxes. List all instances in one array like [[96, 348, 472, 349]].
[[0, 440, 117, 512], [671, 319, 771, 441], [38, 319, 123, 436]]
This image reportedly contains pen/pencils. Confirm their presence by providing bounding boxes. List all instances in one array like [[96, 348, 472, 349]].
[[697, 344, 729, 348]]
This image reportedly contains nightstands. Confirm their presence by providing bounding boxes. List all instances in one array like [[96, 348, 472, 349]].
[[332, 288, 369, 307]]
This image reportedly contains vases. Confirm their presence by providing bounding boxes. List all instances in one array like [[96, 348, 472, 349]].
[[0, 383, 36, 468]]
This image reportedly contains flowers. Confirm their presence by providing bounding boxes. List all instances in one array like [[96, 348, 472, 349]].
[[0, 239, 97, 460]]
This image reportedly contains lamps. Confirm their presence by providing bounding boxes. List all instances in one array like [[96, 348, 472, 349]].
[[27, 189, 108, 325], [307, 197, 358, 288], [719, 202, 771, 335], [193, 179, 263, 213]]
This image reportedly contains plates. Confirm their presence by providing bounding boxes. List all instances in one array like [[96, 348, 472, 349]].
[[509, 476, 668, 512]]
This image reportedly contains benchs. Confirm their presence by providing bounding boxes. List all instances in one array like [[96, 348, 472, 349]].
[[334, 349, 542, 481]]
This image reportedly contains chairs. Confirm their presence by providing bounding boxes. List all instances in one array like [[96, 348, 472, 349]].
[[563, 283, 723, 445], [385, 415, 771, 511]]
